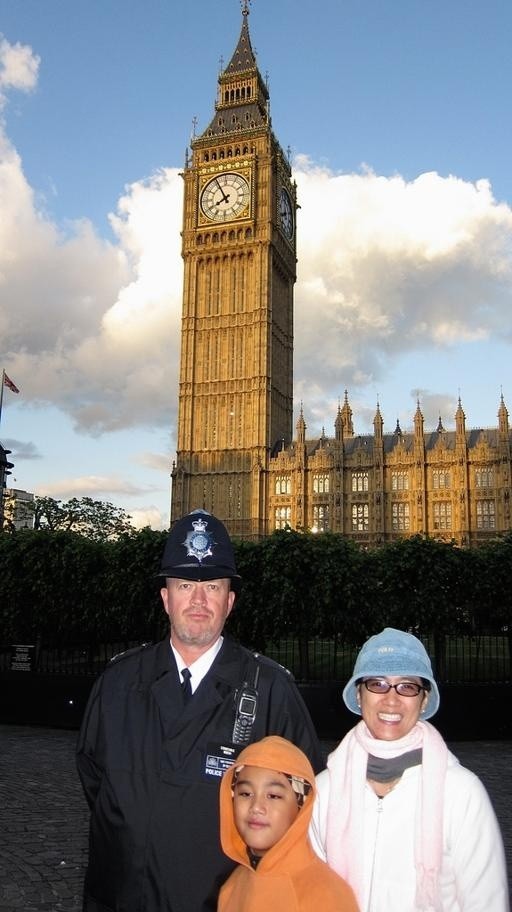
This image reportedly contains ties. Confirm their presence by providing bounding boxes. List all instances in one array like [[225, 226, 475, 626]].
[[180, 669, 195, 699]]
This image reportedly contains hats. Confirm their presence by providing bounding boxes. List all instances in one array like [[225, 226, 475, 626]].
[[152, 513, 243, 584], [341, 626, 441, 723]]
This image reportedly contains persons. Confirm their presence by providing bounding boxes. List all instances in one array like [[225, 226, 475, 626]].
[[205, 732, 359, 910], [309, 625, 512, 912], [74, 508, 323, 910]]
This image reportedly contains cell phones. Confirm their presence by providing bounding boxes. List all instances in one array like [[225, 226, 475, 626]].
[[232, 686, 259, 747]]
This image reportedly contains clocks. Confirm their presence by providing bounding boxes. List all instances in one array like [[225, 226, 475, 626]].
[[191, 162, 259, 228]]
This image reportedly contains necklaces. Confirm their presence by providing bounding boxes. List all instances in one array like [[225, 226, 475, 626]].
[[375, 780, 400, 795]]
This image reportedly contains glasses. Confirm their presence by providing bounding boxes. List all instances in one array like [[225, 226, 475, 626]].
[[359, 678, 428, 698]]
